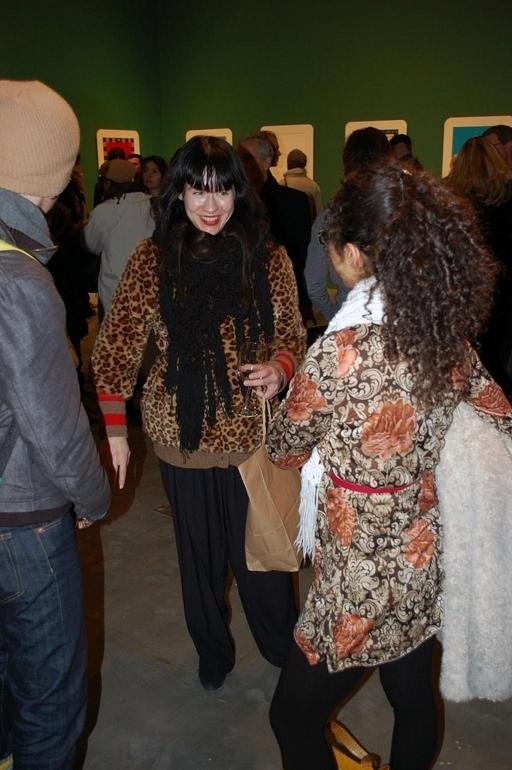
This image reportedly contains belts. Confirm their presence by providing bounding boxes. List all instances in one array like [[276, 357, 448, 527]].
[[329, 468, 415, 494]]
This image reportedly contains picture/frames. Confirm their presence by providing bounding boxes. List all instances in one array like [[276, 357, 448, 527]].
[[344, 119, 408, 147], [96, 128, 141, 171], [259, 124, 315, 185], [442, 115, 512, 179], [184, 128, 234, 148]]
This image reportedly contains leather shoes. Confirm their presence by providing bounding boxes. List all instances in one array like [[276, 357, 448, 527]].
[[197, 654, 234, 690]]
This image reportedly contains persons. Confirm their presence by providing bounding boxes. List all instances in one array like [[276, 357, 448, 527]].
[[91, 136, 309, 690], [2, 80, 108, 770], [43, 126, 512, 446], [267, 156, 512, 769]]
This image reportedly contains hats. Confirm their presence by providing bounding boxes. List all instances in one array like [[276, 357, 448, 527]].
[[0, 79, 81, 199], [286, 149, 307, 166], [102, 159, 137, 181]]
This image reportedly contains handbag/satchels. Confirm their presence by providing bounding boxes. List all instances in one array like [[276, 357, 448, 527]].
[[326, 721, 390, 770], [238, 444, 310, 576]]
